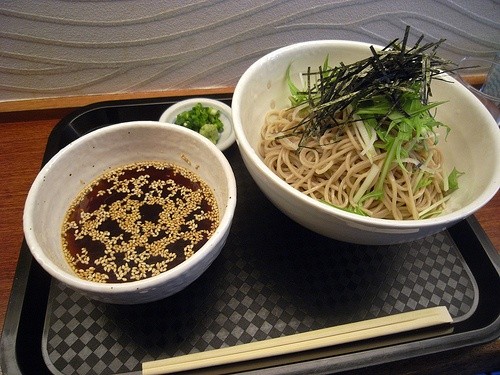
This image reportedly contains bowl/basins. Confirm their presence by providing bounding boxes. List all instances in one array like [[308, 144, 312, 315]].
[[22, 121, 237, 304], [232, 40, 500, 246]]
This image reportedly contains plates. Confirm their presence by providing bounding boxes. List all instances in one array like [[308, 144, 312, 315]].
[[158, 98, 236, 151]]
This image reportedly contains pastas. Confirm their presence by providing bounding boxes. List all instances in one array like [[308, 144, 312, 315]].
[[259, 25, 481, 221]]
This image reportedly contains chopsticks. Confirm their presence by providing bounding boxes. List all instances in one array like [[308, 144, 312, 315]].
[[141, 305, 453, 374]]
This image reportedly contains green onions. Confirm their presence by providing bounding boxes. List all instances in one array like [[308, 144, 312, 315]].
[[174, 102, 225, 146]]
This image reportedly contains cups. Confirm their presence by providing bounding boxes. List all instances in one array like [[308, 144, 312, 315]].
[[455, 56, 500, 126]]
